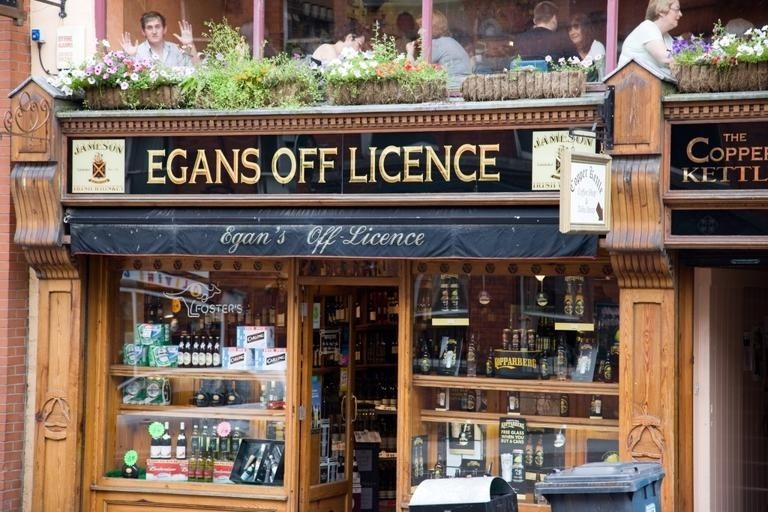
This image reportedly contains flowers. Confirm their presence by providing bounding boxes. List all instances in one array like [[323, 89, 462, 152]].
[[47, 16, 768, 123]]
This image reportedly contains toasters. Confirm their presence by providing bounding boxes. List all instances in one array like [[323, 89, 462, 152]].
[[418, 339, 433, 374]]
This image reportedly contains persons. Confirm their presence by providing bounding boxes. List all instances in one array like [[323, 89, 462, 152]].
[[406, 9, 472, 91], [239, 20, 277, 59], [119, 11, 201, 65], [312, 27, 369, 68], [617, 0, 683, 75], [517, 0, 582, 63], [566, 12, 605, 83]]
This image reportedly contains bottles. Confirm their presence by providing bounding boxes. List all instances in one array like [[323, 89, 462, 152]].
[[563, 282, 585, 323], [326, 291, 398, 325], [434, 454, 442, 479], [121, 461, 139, 478], [604, 352, 613, 382], [314, 333, 397, 367], [525, 432, 545, 468], [194, 380, 241, 406], [259, 381, 278, 409], [146, 294, 164, 323], [191, 424, 242, 461], [149, 418, 172, 459], [227, 302, 275, 328], [175, 421, 187, 460], [177, 334, 220, 367], [419, 293, 433, 323], [188, 450, 213, 482], [485, 345, 493, 376]]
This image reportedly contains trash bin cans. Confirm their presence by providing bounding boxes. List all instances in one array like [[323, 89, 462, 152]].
[[534, 462, 666, 512], [408, 477, 518, 512]]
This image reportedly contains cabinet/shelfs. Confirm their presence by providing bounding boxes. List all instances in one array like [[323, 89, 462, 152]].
[[103, 259, 620, 512]]
